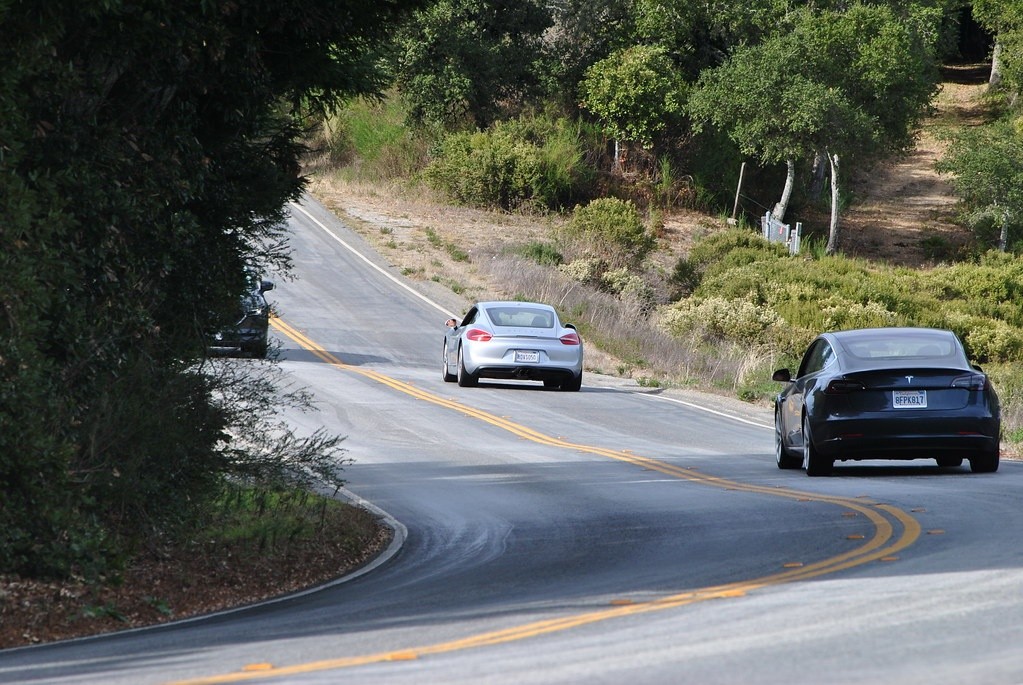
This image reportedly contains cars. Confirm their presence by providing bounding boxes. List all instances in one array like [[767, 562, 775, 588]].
[[772, 327, 1000, 476], [443, 301, 583, 392], [208, 263, 273, 358]]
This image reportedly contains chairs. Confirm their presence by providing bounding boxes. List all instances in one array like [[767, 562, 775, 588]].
[[532, 316, 549, 328], [917, 346, 941, 356], [850, 346, 870, 357]]
